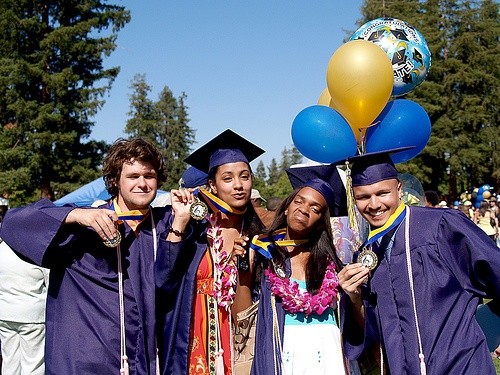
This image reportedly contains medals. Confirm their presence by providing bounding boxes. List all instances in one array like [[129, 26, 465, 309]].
[[271, 259, 286, 279], [103, 230, 121, 247], [357, 247, 378, 270], [190, 197, 208, 220]]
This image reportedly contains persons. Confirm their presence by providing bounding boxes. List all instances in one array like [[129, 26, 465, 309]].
[[331, 147, 500, 375], [0, 137, 359, 375], [425, 188, 500, 248]]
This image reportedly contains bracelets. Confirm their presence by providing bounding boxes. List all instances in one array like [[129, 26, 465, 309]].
[[167, 225, 186, 238]]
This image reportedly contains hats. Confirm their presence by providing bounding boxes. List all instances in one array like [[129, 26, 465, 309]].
[[180, 166, 210, 187], [454, 184, 500, 208], [251, 189, 268, 203], [285, 165, 349, 217], [331, 145, 418, 234], [439, 201, 447, 205], [182, 128, 267, 174]]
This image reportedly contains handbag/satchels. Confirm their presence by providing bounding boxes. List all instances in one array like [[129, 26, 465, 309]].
[[490, 217, 496, 227]]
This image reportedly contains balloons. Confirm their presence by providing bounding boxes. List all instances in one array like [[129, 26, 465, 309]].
[[291, 18, 432, 164], [475, 185, 492, 207]]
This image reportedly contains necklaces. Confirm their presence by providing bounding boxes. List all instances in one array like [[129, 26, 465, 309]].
[[205, 213, 238, 311], [265, 262, 339, 315]]
